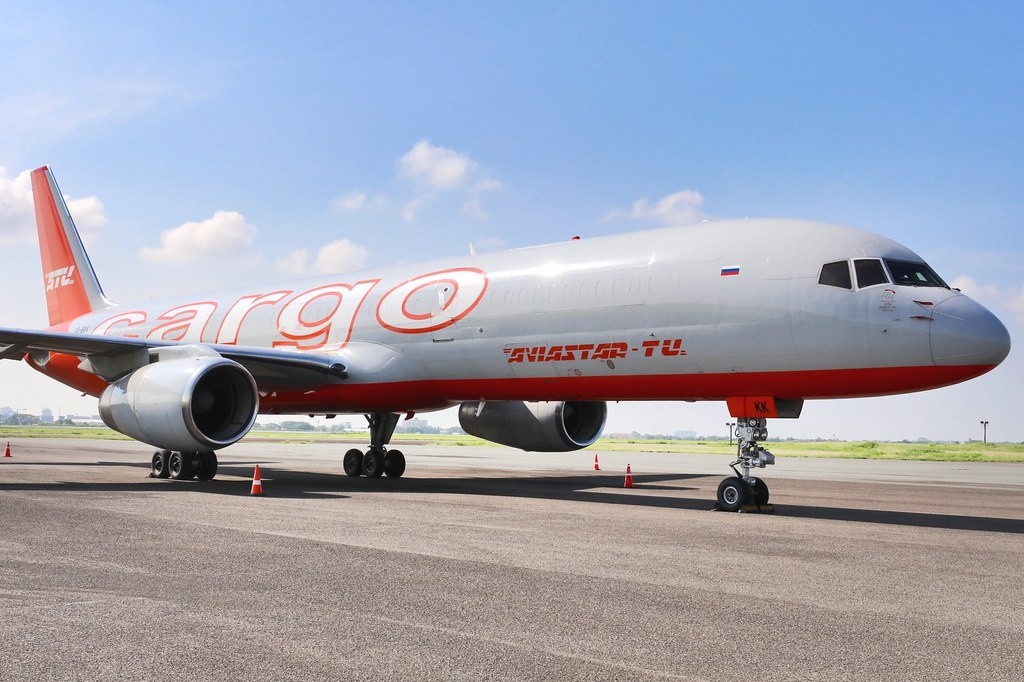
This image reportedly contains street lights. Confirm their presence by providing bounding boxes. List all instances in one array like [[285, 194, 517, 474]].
[[726, 422, 735, 446], [981, 420, 988, 443]]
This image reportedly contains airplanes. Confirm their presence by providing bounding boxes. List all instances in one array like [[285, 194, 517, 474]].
[[0, 162, 1012, 513]]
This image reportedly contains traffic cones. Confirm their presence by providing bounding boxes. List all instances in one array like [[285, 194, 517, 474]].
[[593, 453, 602, 471], [2, 441, 13, 457], [246, 464, 266, 497], [622, 463, 636, 488]]
